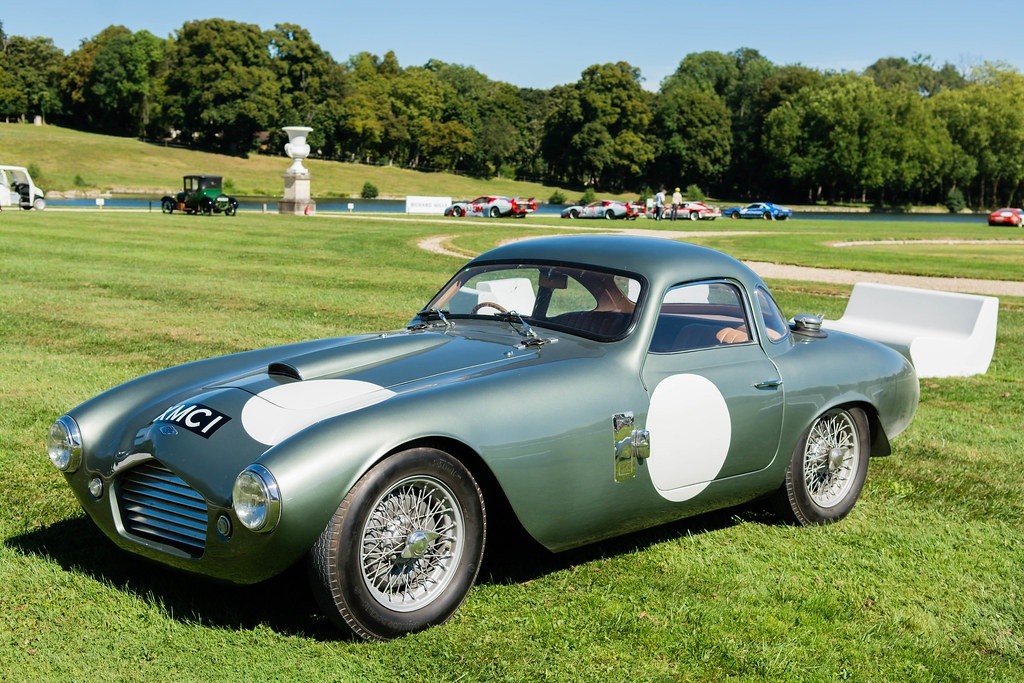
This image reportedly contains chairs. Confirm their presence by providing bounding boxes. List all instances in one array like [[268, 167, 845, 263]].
[[576, 310, 624, 338], [669, 324, 711, 349]]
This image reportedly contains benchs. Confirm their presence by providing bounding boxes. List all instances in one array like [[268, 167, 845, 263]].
[[550, 310, 750, 355], [792, 283, 1000, 379]]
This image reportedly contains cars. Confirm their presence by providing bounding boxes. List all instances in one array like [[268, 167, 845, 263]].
[[47, 233, 920, 645], [160, 174, 239, 216], [989, 207, 1024, 228]]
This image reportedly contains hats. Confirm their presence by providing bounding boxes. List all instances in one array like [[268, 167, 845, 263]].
[[675, 187, 680, 191]]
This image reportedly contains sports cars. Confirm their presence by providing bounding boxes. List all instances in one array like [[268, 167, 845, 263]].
[[561, 199, 640, 221], [649, 200, 723, 221], [722, 201, 794, 221], [444, 195, 537, 219]]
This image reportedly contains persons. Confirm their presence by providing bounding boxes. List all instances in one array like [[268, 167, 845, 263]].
[[654, 189, 668, 221], [670, 187, 682, 221]]
